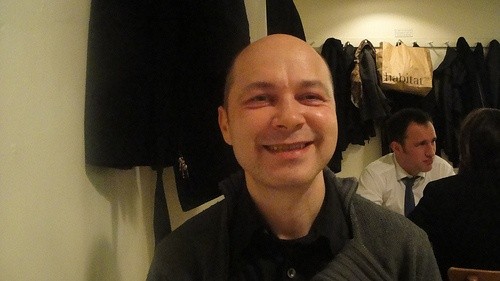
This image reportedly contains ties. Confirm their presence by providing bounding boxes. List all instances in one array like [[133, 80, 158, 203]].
[[402, 176, 420, 217]]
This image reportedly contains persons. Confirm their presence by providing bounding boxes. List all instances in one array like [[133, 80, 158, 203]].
[[356, 108, 456, 217], [409, 108, 500, 281], [146, 34, 441, 281]]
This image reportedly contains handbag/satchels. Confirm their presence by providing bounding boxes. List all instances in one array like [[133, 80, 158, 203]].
[[377, 40, 433, 96]]
